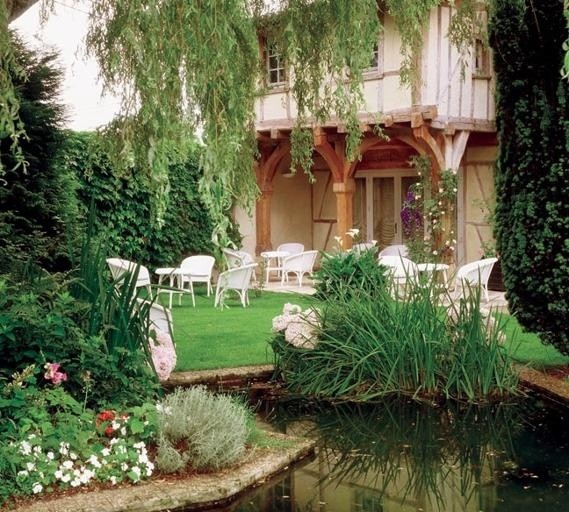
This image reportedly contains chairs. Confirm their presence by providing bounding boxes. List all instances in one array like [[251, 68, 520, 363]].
[[221, 247, 257, 283], [106, 258, 153, 301], [377, 256, 419, 295], [453, 256, 497, 303], [179, 255, 216, 296], [214, 262, 259, 308], [380, 244, 410, 258], [277, 243, 304, 277], [280, 250, 319, 287]]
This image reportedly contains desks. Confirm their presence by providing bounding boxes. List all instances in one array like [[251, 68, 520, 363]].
[[261, 251, 289, 283], [154, 268, 197, 309], [416, 262, 450, 288]]
